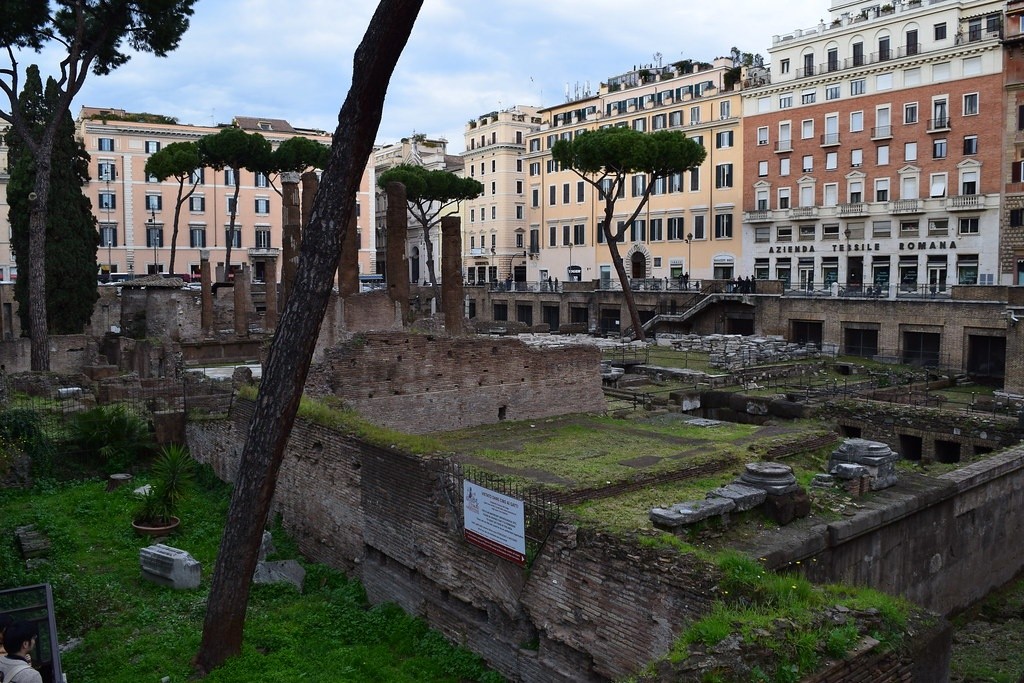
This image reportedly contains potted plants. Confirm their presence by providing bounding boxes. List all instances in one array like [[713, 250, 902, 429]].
[[131, 441, 201, 538]]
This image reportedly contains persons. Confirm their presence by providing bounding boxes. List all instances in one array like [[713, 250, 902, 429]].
[[694, 281, 699, 291], [738, 274, 756, 294], [548, 276, 554, 291], [506, 273, 511, 290], [493, 276, 498, 287], [679, 271, 688, 291], [867, 286, 873, 297], [0, 619, 43, 683], [555, 277, 558, 292], [931, 283, 936, 299], [831, 280, 839, 297], [873, 282, 882, 298], [664, 277, 668, 283]]
